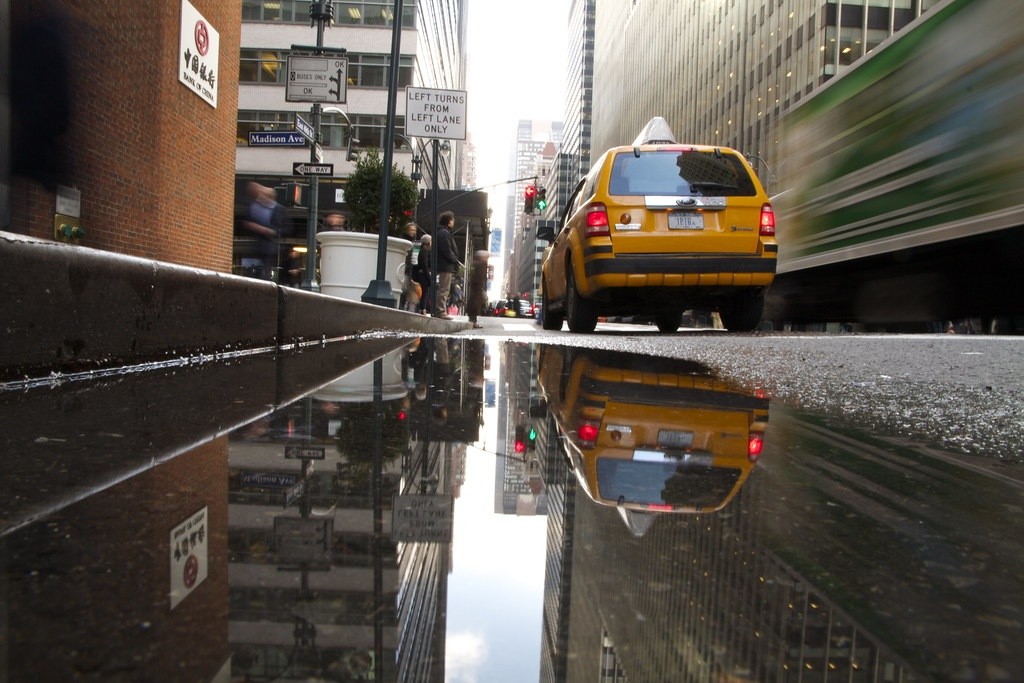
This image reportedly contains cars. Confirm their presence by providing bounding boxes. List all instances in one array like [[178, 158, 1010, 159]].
[[534, 343, 769, 537], [492, 299, 542, 318], [537, 117, 778, 335]]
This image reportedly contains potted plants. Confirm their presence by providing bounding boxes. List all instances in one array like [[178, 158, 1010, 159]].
[[316, 146, 421, 309], [314, 346, 414, 471]]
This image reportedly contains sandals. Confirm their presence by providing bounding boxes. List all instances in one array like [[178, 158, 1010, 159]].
[[474, 325, 483, 328]]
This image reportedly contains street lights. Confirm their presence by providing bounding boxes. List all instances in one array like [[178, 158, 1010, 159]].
[[395, 132, 453, 283]]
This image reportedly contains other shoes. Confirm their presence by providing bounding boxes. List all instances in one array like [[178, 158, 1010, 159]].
[[438, 311, 453, 320]]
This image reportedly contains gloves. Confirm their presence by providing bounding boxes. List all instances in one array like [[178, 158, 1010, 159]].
[[427, 278, 430, 286]]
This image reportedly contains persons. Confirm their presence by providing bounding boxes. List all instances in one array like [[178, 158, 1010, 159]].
[[327, 213, 345, 231], [414, 337, 454, 425], [513, 292, 521, 317], [466, 250, 490, 328], [244, 181, 284, 281], [404, 211, 463, 319]]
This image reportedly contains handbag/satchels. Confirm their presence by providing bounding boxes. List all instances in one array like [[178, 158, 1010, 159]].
[[481, 290, 488, 301], [413, 264, 428, 281]]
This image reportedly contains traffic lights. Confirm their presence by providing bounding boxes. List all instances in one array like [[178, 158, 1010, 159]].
[[345, 136, 360, 162], [292, 161, 335, 177], [526, 187, 536, 216], [538, 187, 547, 209]]
